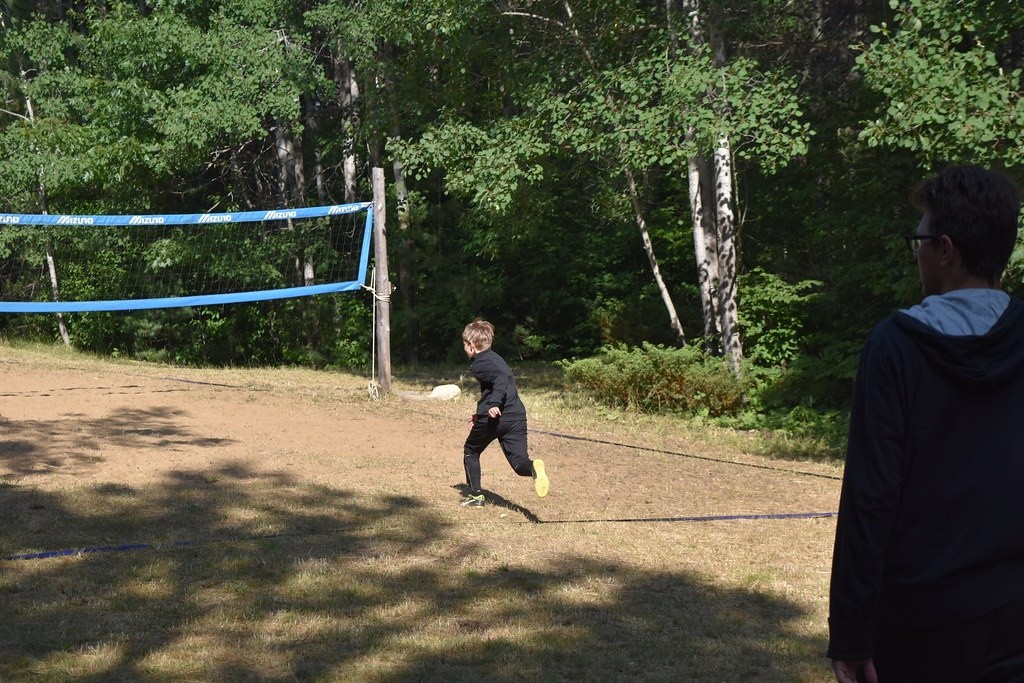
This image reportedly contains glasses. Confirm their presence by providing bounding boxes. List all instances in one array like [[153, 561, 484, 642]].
[[904, 232, 940, 252]]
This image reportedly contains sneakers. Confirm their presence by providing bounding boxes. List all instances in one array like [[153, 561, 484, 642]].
[[461, 494, 486, 509], [532, 458, 550, 497]]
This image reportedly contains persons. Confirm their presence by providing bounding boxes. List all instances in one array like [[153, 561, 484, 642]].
[[457, 320, 550, 510], [823, 164, 1024, 682]]
